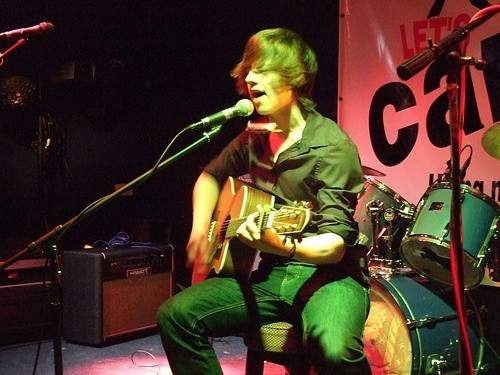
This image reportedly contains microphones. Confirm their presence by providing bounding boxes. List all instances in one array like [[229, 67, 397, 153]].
[[0, 22, 54, 39], [189, 99, 254, 131]]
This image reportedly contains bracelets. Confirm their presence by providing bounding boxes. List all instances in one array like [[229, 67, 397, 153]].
[[289, 243, 296, 259]]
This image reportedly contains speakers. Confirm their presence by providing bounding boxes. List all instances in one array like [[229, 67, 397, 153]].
[[0, 257, 60, 349], [60, 243, 173, 347]]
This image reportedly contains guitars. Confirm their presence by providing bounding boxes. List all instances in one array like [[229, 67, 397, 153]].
[[189, 174, 315, 285]]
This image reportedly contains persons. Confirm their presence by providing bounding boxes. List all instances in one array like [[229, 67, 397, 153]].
[[156, 28, 371, 375]]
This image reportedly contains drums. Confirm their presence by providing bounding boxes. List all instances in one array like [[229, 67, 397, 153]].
[[353, 175, 418, 261], [363, 267, 487, 375], [398, 180, 500, 292]]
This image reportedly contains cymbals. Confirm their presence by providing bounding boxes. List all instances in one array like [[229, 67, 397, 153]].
[[481, 118, 500, 162], [361, 164, 388, 178]]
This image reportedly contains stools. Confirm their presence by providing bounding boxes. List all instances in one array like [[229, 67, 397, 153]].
[[242, 319, 306, 375]]
